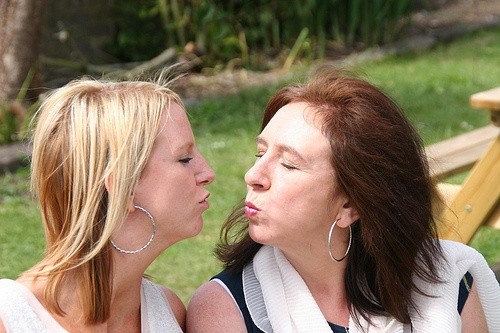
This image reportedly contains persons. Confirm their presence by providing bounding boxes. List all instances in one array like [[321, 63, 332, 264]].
[[183, 63, 500, 332], [0, 75, 216, 333]]
